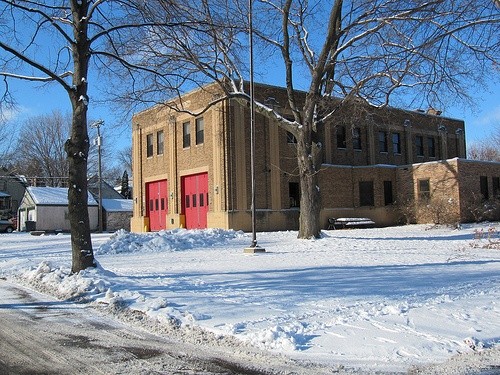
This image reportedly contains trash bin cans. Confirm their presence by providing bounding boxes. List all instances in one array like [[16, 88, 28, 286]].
[[24, 221, 36, 232]]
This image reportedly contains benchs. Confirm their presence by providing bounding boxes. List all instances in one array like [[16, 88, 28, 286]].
[[327, 217, 376, 231]]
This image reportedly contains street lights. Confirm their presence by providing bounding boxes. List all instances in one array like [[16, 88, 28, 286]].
[[91, 121, 105, 232]]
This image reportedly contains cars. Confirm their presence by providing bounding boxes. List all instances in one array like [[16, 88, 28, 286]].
[[1, 220, 15, 233]]
